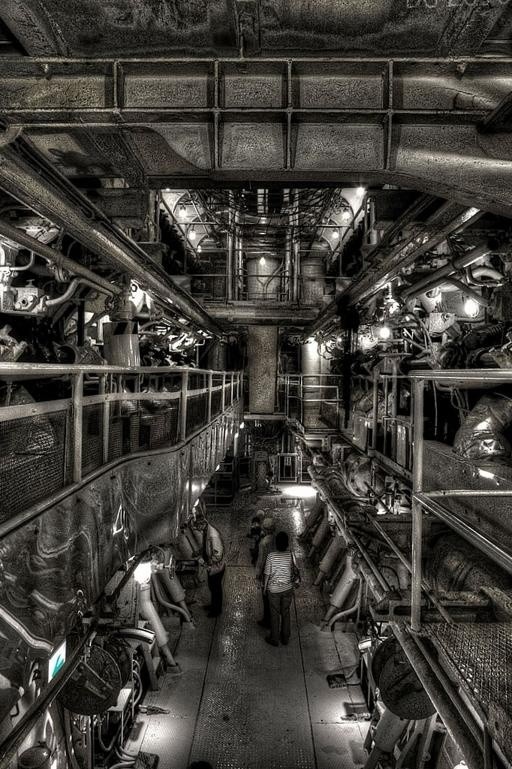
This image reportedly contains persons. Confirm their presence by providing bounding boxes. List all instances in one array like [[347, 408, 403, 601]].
[[245, 507, 266, 566], [253, 516, 276, 631], [190, 514, 226, 617], [263, 531, 292, 647]]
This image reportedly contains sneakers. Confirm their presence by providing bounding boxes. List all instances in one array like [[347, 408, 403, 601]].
[[202, 603, 222, 617], [257, 620, 291, 646]]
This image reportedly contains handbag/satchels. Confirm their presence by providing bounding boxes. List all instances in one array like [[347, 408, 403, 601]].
[[291, 566, 301, 588]]
